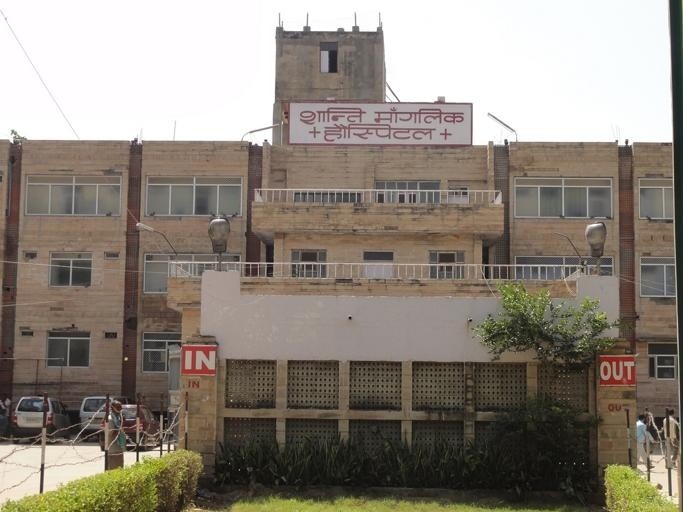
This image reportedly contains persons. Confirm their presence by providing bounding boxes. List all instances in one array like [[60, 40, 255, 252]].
[[103, 401, 125, 472], [663, 408, 680, 468], [635, 414, 656, 469], [0, 393, 12, 441]]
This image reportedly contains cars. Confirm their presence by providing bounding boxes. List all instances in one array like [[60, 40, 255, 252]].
[[99, 404, 159, 451]]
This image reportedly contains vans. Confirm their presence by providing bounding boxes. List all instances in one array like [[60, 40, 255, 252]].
[[79, 396, 134, 433], [14, 396, 72, 437]]
